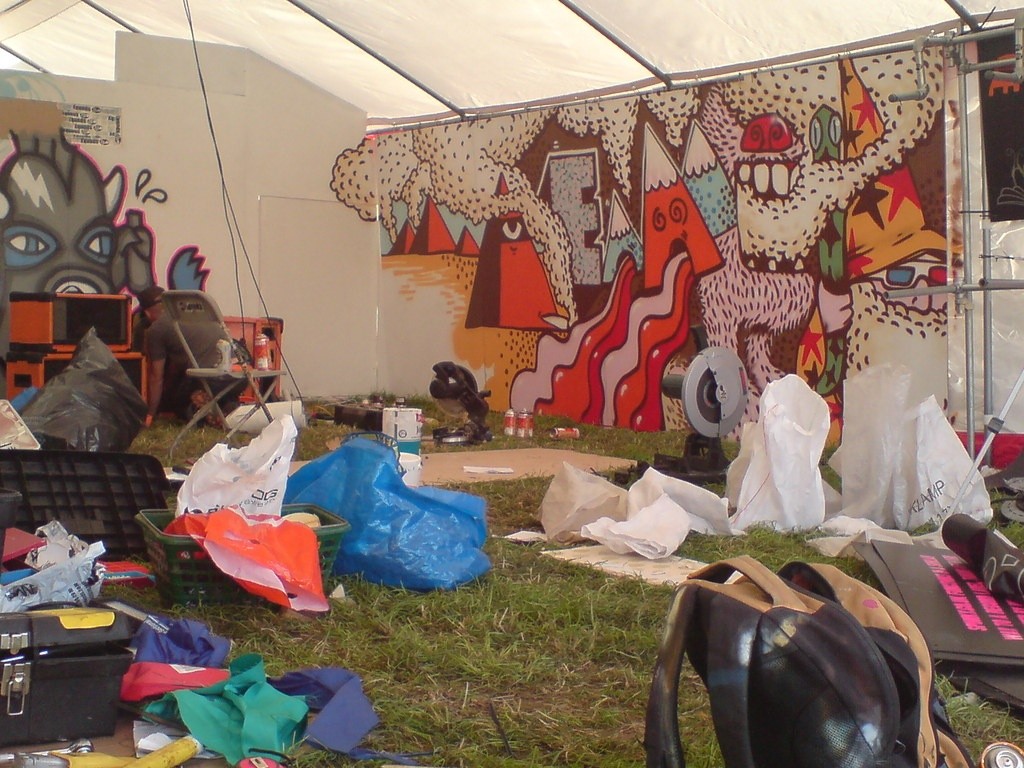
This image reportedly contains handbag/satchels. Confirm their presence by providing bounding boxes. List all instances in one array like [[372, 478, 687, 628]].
[[174, 416, 298, 520], [161, 510, 329, 614]]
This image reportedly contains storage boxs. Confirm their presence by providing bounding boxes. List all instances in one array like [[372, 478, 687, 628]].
[[0, 606, 135, 749], [134, 503, 352, 611]]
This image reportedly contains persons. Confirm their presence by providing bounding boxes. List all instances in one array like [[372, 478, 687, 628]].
[[139, 286, 246, 429]]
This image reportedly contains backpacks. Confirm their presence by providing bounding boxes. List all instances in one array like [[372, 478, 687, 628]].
[[642, 556, 975, 768]]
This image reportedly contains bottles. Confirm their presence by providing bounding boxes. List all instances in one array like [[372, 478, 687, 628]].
[[504, 407, 515, 436], [527, 411, 534, 438], [254, 329, 271, 369], [550, 426, 581, 440], [516, 408, 527, 437]]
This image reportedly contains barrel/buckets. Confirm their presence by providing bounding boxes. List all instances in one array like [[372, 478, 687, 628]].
[[382, 407, 422, 457], [395, 451, 422, 490]]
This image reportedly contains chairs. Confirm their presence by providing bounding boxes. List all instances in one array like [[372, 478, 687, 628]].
[[162, 289, 285, 463]]
